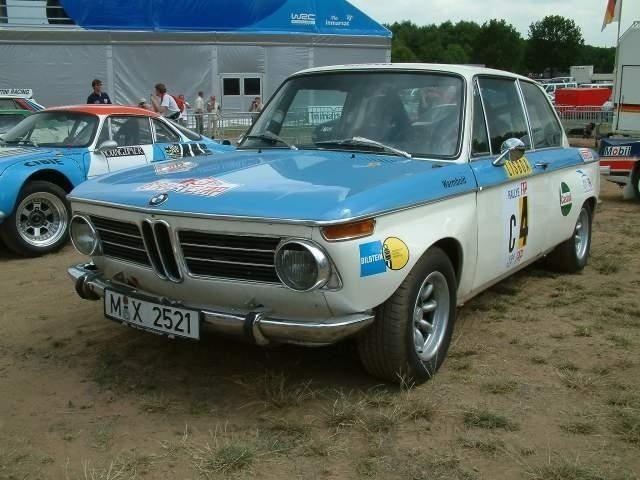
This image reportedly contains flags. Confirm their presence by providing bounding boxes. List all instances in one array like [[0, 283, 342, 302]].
[[601, 0, 623, 33]]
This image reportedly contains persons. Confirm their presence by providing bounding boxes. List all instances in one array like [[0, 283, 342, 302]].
[[87, 79, 112, 105], [206, 95, 222, 139], [193, 91, 205, 136], [250, 97, 264, 125], [137, 83, 190, 130]]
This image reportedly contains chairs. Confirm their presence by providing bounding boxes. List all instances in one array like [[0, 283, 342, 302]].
[[350, 94, 410, 143]]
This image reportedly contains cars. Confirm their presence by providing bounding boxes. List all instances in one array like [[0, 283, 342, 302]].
[[0, 104, 234, 259], [0, 88, 89, 148], [65, 61, 603, 388]]
